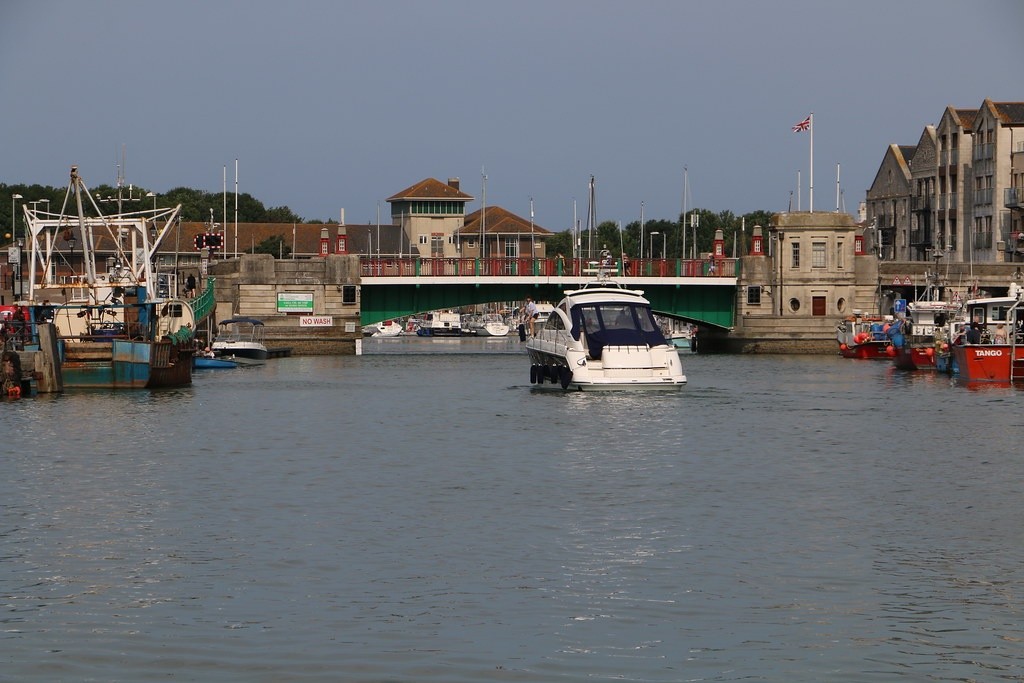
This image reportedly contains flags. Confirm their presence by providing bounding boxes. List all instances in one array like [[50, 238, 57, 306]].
[[792, 115, 810, 133], [972, 280, 979, 295]]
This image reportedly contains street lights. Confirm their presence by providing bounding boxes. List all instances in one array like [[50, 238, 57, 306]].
[[38, 198, 51, 220], [12, 194, 23, 248], [649, 231, 659, 260], [145, 191, 157, 229]]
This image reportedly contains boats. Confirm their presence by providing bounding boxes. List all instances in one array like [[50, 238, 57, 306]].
[[192, 339, 240, 369], [21, 166, 216, 398], [661, 326, 696, 350], [210, 314, 269, 368], [829, 226, 1024, 391], [522, 274, 688, 394], [364, 301, 557, 340]]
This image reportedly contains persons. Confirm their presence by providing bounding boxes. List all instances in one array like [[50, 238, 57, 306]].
[[957, 323, 978, 344], [518, 310, 528, 343], [706, 252, 716, 277], [526, 298, 539, 340], [993, 323, 1007, 344], [971, 316, 983, 344], [688, 325, 698, 353], [655, 315, 668, 336], [556, 252, 567, 275], [973, 313, 982, 322], [187, 273, 196, 299], [617, 253, 632, 277], [4, 304, 27, 351], [615, 309, 630, 326], [600, 243, 610, 257]]
